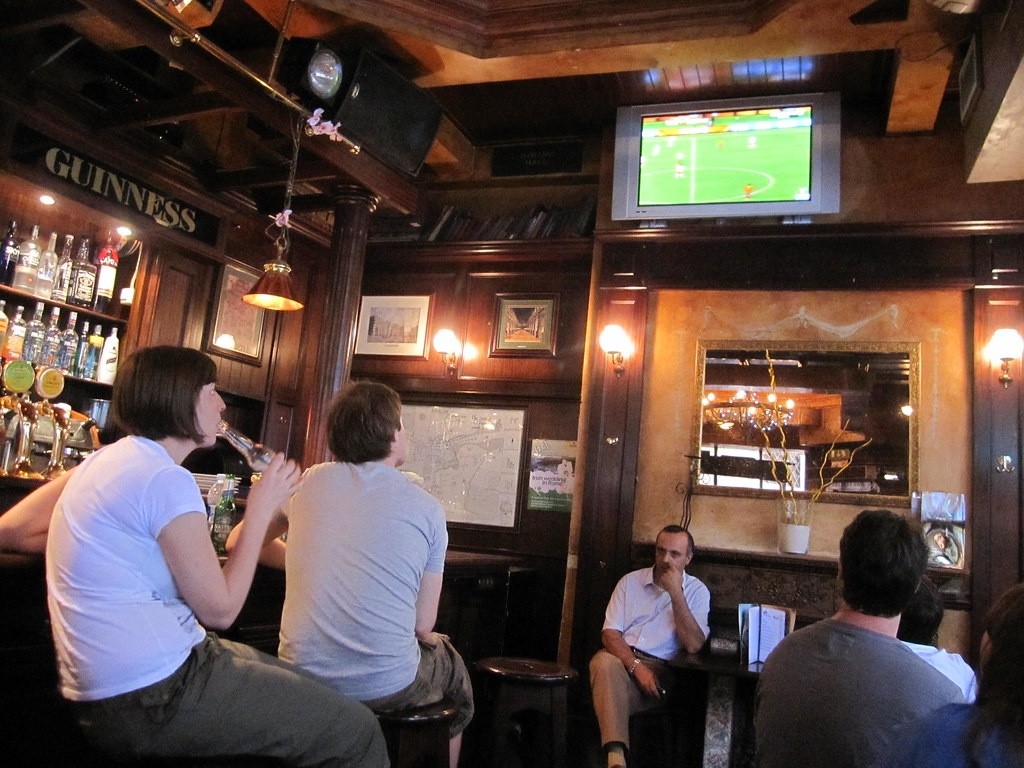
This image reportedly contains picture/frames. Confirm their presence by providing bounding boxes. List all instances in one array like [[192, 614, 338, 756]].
[[486, 290, 560, 358], [204, 264, 268, 367], [351, 291, 437, 360]]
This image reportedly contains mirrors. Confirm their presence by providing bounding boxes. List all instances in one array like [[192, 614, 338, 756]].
[[689, 339, 921, 507]]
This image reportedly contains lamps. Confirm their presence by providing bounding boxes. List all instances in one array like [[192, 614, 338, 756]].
[[431, 329, 463, 371], [599, 324, 633, 375], [699, 359, 793, 432], [984, 329, 1024, 387], [241, 113, 311, 310]]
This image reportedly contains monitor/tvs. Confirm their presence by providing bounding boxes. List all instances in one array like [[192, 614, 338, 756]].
[[611, 91, 840, 220], [329, 44, 446, 176]]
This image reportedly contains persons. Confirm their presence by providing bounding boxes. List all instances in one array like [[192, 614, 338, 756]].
[[874, 581, 1024, 768], [1, 344, 389, 768], [743, 183, 752, 201], [225, 380, 475, 768], [750, 509, 962, 767], [589, 525, 711, 768], [896, 572, 978, 704]]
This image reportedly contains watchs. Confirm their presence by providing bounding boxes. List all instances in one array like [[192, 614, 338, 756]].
[[627, 659, 640, 677]]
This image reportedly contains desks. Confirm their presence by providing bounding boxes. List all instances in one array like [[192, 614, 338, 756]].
[[668, 647, 760, 768]]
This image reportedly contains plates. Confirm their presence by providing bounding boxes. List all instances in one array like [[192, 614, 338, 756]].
[[191, 472, 243, 496]]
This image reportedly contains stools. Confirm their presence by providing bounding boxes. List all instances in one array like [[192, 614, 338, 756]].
[[473, 657, 576, 768], [375, 703, 457, 768]]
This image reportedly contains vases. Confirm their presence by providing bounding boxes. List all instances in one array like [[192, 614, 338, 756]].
[[778, 523, 812, 554]]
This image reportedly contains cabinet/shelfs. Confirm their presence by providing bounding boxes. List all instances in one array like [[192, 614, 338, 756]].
[[1, 285, 127, 492]]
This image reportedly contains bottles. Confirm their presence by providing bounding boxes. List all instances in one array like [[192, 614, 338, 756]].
[[34, 231, 60, 299], [13, 224, 42, 294], [0, 296, 120, 385], [208, 473, 236, 554], [218, 419, 286, 475], [91, 226, 119, 314], [66, 236, 98, 309], [0, 220, 20, 287], [52, 235, 75, 302]]
